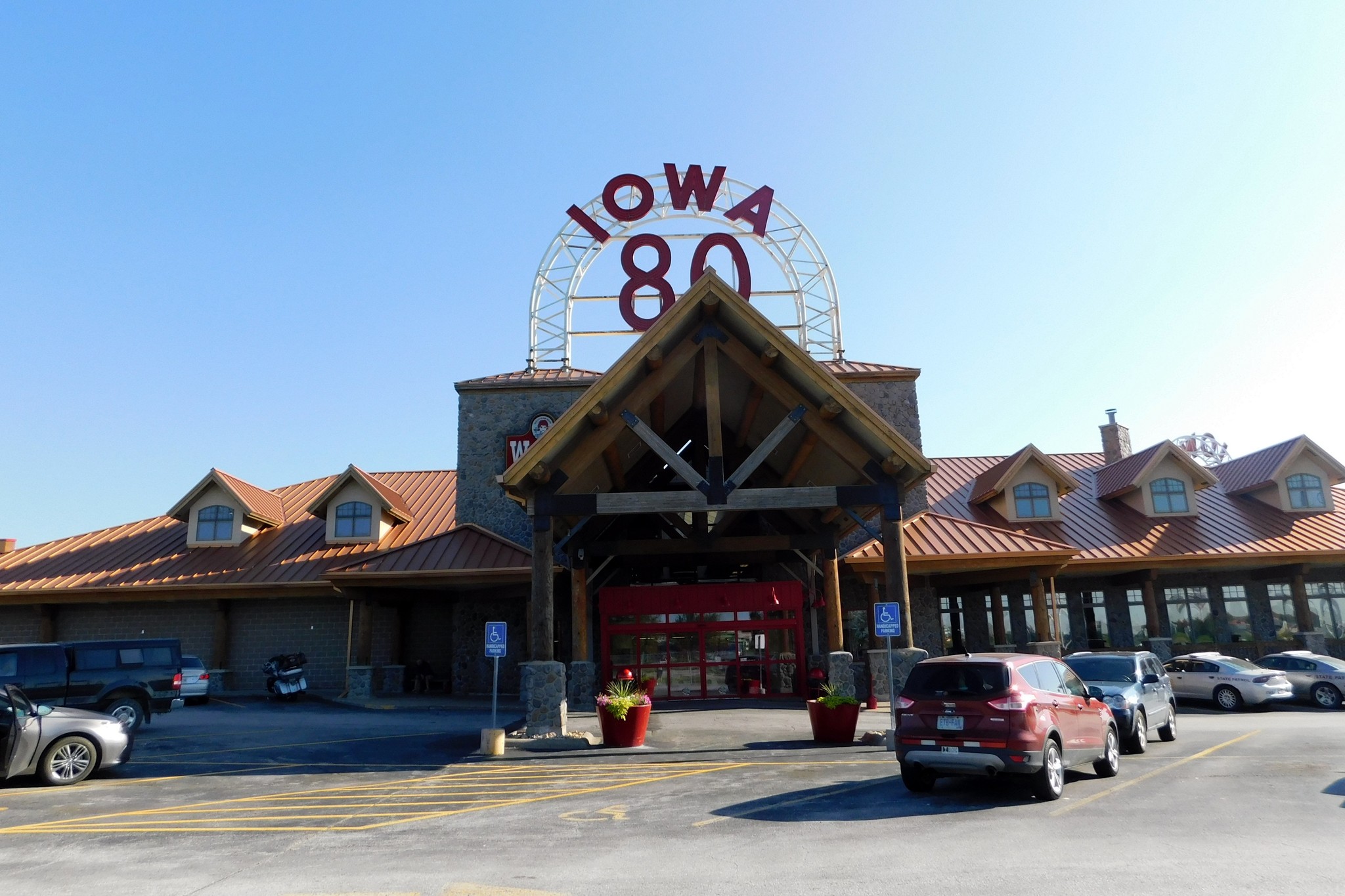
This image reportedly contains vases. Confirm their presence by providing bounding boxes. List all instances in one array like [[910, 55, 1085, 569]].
[[593, 703, 653, 747]]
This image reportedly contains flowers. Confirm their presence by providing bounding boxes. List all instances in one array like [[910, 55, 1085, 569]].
[[595, 677, 651, 721]]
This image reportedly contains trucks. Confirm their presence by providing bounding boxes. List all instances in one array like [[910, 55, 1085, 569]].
[[0, 636, 183, 736]]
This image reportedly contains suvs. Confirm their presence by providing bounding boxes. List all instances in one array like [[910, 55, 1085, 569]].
[[1058, 648, 1177, 755], [893, 650, 1122, 792], [725, 654, 766, 694]]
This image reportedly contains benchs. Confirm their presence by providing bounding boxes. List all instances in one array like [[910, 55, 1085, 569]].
[[412, 678, 450, 694]]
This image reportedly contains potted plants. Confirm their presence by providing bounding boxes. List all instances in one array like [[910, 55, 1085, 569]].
[[805, 679, 863, 744]]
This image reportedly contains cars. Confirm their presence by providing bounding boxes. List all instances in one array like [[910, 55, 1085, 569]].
[[1245, 651, 1345, 710], [179, 654, 210, 704], [0, 681, 132, 786], [1154, 650, 1296, 711]]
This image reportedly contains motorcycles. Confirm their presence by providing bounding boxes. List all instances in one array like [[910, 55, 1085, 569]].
[[260, 652, 310, 701]]
